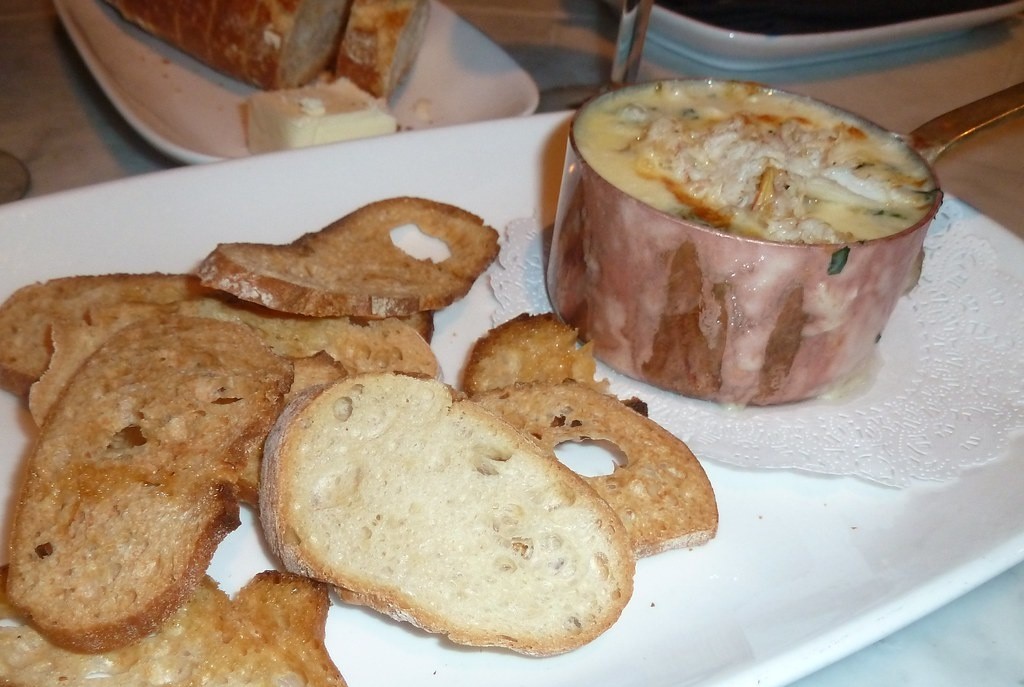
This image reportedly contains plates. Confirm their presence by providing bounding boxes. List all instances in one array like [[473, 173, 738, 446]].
[[608, 1, 1024, 71], [0, 108, 1023, 687], [52, 0, 542, 166]]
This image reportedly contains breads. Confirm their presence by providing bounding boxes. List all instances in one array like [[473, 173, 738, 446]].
[[0, 196, 721, 687], [106, 0, 435, 104], [241, 76, 398, 153]]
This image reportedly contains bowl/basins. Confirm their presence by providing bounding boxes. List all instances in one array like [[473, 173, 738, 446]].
[[543, 75, 941, 410]]
